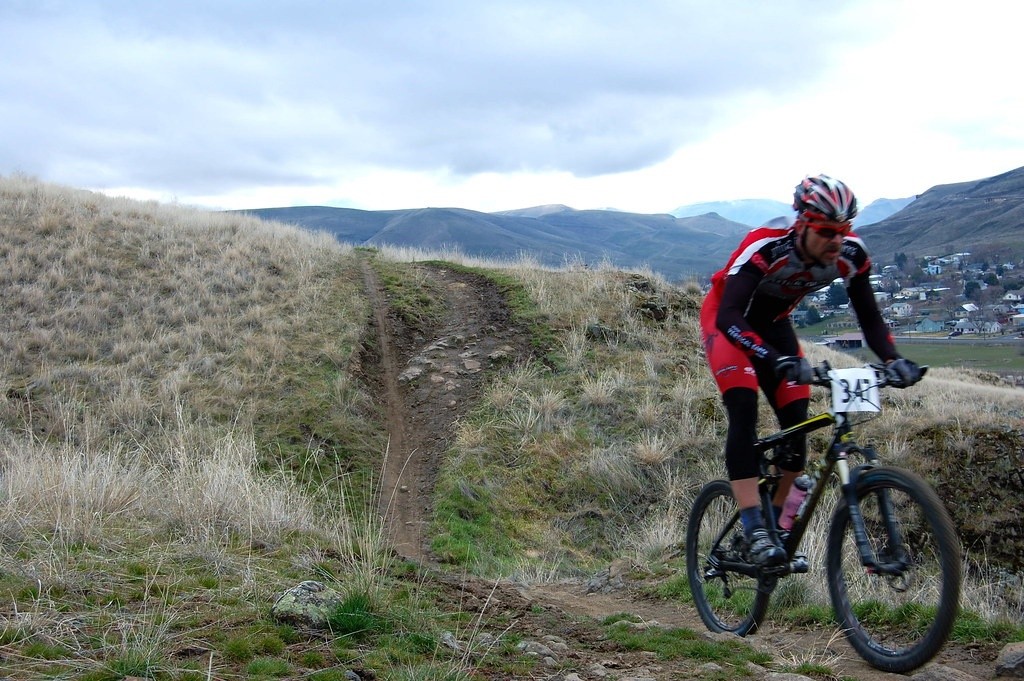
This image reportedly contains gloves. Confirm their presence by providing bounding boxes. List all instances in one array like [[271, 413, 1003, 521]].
[[885, 359, 923, 388], [773, 355, 815, 386]]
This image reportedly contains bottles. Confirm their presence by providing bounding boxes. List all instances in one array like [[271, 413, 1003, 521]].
[[778, 474, 811, 530]]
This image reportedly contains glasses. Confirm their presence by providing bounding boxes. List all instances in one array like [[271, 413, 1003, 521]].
[[809, 223, 854, 240]]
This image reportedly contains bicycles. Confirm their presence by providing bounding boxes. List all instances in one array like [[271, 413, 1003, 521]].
[[686, 359, 963, 673]]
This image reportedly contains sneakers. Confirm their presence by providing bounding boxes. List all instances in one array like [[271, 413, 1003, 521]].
[[741, 523, 785, 566], [768, 515, 809, 573]]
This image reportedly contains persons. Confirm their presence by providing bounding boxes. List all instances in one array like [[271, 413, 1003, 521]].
[[700, 173, 925, 576]]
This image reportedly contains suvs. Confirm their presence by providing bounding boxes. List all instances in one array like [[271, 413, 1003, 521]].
[[949, 331, 962, 336]]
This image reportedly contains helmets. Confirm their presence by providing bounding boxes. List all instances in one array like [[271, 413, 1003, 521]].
[[791, 174, 859, 222]]
